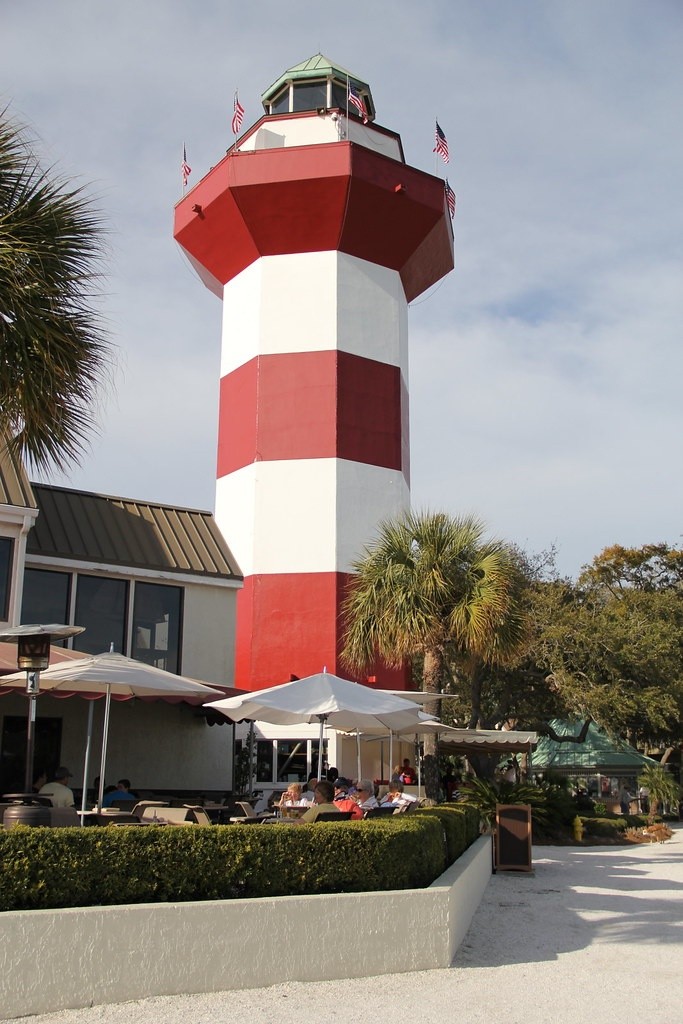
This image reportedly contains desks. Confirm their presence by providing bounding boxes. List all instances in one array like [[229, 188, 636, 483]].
[[79, 810, 140, 828], [204, 806, 228, 824]]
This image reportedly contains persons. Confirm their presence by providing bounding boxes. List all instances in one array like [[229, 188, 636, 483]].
[[30, 766, 46, 795], [100, 784, 136, 807], [273, 738, 486, 818], [116, 779, 131, 793], [86, 776, 106, 805], [39, 767, 76, 810], [572, 776, 653, 819]]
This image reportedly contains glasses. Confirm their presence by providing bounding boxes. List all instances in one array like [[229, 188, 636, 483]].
[[356, 788, 364, 792]]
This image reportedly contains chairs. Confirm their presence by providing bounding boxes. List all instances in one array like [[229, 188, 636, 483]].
[[0, 790, 427, 827]]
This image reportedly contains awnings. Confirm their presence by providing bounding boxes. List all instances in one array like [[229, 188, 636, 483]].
[[417, 723, 540, 754], [0, 640, 278, 728]]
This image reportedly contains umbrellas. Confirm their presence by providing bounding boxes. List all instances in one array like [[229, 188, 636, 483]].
[[202, 666, 459, 803], [0, 643, 225, 812]]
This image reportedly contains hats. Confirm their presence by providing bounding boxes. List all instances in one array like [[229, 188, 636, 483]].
[[54, 766, 73, 779], [334, 777, 349, 786]]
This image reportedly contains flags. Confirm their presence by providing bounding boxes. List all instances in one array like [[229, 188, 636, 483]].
[[348, 81, 369, 124], [445, 179, 456, 219], [231, 93, 244, 135], [432, 120, 450, 163], [181, 143, 191, 186]]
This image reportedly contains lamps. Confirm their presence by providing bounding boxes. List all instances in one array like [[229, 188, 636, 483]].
[[317, 107, 328, 115]]
